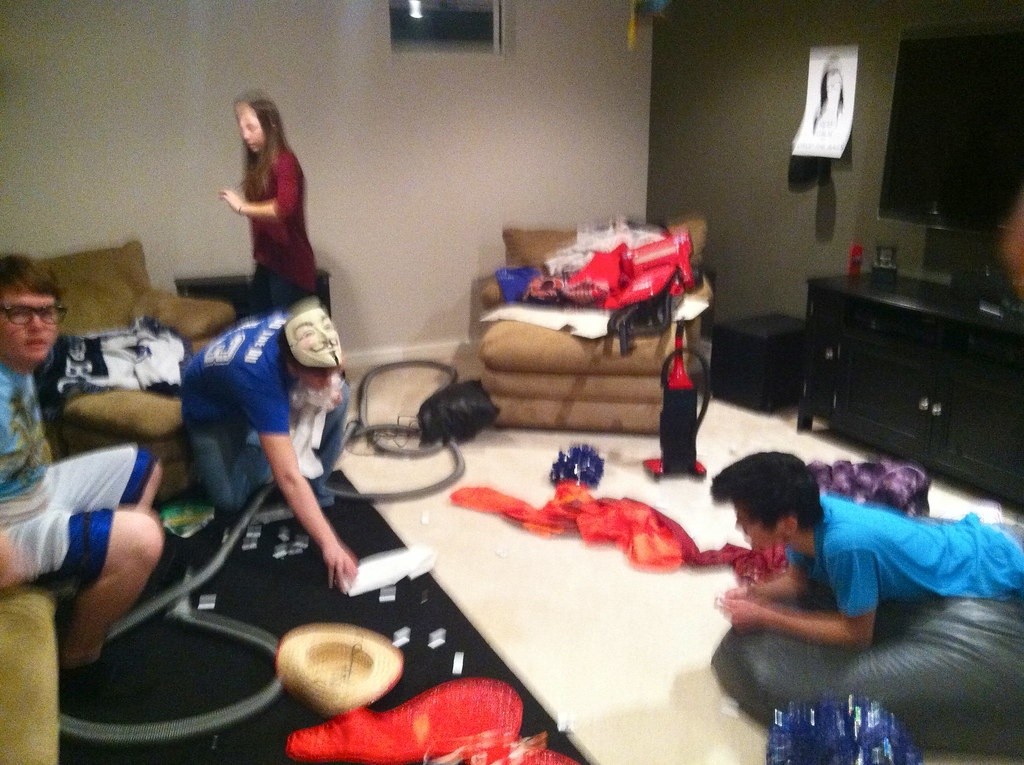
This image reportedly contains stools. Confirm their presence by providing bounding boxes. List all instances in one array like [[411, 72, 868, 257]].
[[712, 313, 805, 415]]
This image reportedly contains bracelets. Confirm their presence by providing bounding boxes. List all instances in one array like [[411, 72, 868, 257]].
[[239, 201, 245, 217]]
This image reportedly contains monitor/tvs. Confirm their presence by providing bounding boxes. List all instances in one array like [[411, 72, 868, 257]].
[[875, 36, 1024, 233]]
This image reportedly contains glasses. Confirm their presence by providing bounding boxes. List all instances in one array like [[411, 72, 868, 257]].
[[0, 302, 68, 325]]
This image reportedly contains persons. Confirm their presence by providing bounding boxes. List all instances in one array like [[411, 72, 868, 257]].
[[182, 307, 360, 597], [0, 252, 165, 675], [217, 91, 317, 322], [710, 450, 1023, 650]]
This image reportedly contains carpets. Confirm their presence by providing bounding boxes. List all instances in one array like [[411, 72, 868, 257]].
[[57, 469, 591, 765]]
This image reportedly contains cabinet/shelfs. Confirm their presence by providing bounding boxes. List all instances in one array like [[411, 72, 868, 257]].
[[798, 272, 1023, 505]]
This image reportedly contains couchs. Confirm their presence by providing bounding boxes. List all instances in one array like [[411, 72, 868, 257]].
[[25, 240, 236, 503], [0, 587, 58, 763], [478, 223, 712, 436]]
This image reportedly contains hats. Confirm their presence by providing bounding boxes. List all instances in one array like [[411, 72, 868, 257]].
[[276, 621, 401, 717]]
